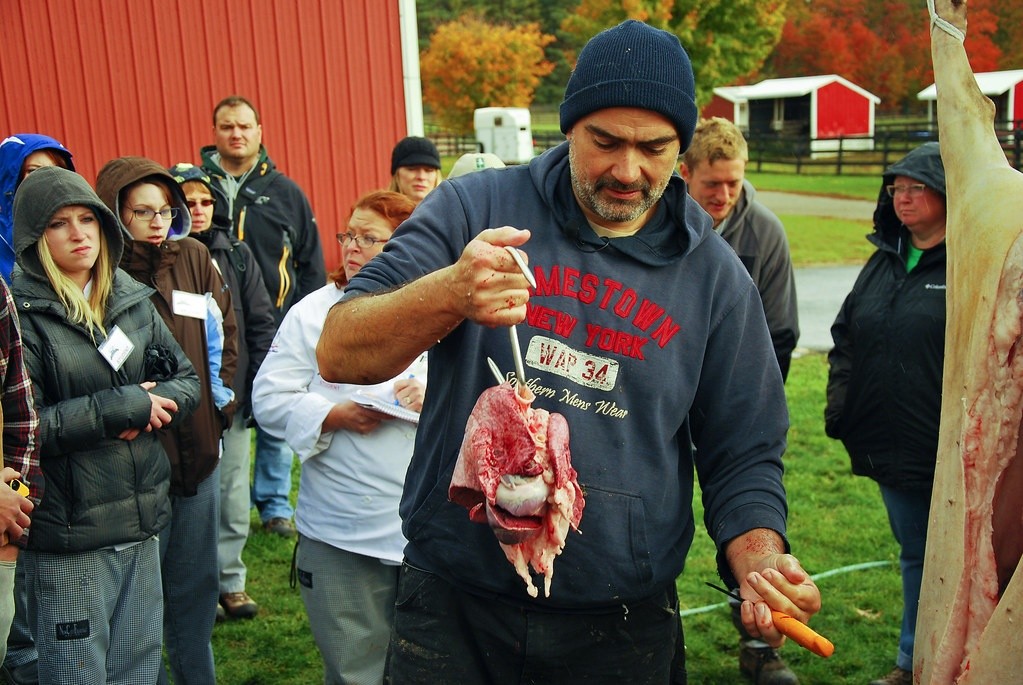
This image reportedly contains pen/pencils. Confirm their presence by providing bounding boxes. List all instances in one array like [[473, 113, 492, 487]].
[[393, 355, 425, 407]]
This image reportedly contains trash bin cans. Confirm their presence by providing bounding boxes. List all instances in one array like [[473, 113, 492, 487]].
[[472, 105, 534, 164]]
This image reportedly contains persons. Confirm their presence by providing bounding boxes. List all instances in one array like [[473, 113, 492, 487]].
[[388, 136, 443, 206], [447, 153, 507, 181], [825, 141, 947, 685], [678, 114, 800, 685], [252, 190, 428, 685], [317, 17, 821, 685], [0, 132, 278, 685], [197, 95, 327, 538]]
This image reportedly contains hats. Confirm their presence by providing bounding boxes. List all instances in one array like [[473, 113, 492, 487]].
[[448, 152, 510, 179], [391, 137, 441, 176], [559, 19, 698, 155]]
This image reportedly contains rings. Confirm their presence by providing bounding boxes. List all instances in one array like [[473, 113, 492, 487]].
[[404, 397, 411, 404]]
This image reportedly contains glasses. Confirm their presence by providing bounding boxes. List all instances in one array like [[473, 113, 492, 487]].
[[123, 204, 180, 220], [335, 232, 389, 249], [186, 199, 217, 208], [887, 184, 926, 198]]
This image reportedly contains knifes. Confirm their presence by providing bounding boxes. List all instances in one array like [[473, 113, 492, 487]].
[[703, 580, 835, 659]]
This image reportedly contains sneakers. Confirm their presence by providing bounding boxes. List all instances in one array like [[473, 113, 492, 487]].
[[218, 592, 258, 618], [869, 667, 913, 685], [263, 517, 296, 538], [739, 647, 800, 685]]
